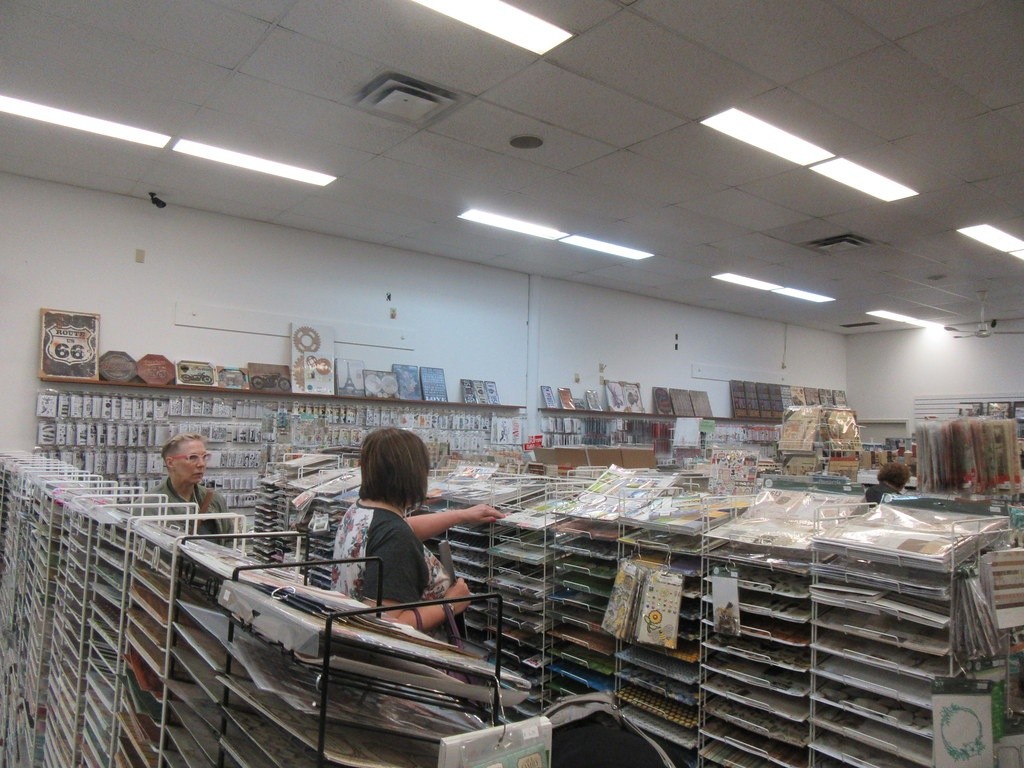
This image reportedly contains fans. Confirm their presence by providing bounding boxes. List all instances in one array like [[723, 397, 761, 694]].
[[944, 291, 1024, 339]]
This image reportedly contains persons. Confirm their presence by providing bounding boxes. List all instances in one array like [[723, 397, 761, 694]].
[[330, 428, 506, 632], [848, 460, 912, 519], [132, 432, 232, 546]]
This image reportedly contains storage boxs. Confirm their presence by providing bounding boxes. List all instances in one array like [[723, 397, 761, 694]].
[[174, 359, 250, 390]]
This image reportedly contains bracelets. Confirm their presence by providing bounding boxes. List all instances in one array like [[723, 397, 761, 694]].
[[440, 603, 455, 618]]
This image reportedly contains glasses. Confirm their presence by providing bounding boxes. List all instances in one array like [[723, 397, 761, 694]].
[[173, 453, 212, 464]]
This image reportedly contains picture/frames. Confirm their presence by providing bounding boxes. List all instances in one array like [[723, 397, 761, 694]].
[[37, 308, 100, 382]]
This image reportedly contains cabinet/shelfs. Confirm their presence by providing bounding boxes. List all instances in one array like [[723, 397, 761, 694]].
[[0, 448, 1013, 768]]
